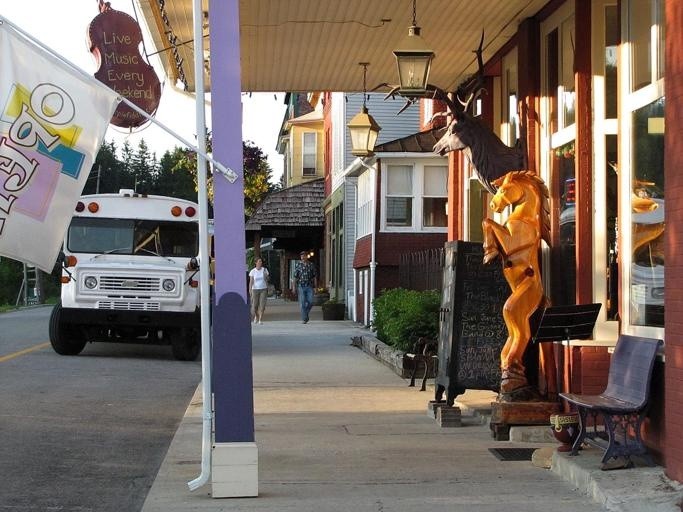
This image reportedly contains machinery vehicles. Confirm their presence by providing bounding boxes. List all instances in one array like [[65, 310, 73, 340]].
[[558, 198, 666, 317], [49, 188, 214, 360]]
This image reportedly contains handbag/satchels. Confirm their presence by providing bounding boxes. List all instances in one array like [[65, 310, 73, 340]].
[[267, 282, 273, 297]]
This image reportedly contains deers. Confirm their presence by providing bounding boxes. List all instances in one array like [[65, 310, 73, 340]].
[[370, 28, 524, 196]]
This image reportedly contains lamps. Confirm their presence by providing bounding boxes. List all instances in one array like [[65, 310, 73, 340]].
[[346, 63, 382, 161], [391, 1, 436, 104]]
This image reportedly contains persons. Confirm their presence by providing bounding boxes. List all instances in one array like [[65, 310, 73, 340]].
[[291, 251, 318, 324], [248, 256, 270, 325]]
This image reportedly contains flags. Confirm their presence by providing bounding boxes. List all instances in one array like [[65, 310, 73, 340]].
[[0, 19, 122, 276]]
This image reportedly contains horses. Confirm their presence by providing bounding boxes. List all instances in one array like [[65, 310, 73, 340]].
[[480, 168, 553, 396]]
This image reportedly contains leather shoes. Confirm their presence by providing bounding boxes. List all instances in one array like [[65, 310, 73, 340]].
[[303, 318, 309, 324], [258, 321, 263, 325], [253, 319, 258, 323]]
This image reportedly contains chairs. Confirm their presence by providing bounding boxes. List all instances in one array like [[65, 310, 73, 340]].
[[559, 334, 662, 469]]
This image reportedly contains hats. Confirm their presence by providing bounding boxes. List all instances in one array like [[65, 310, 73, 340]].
[[299, 251, 307, 255]]
